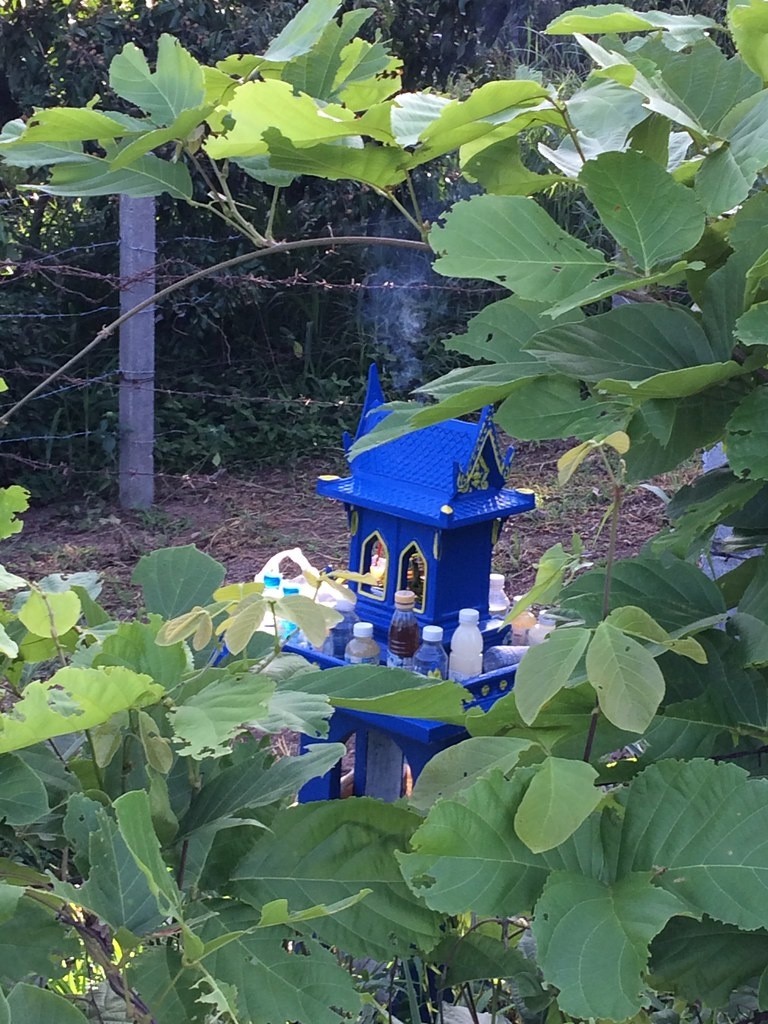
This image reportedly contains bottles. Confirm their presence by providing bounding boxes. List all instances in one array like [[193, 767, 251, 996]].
[[278, 586, 301, 642], [510, 595, 536, 646], [413, 625, 448, 681], [448, 608, 483, 683], [345, 622, 381, 667], [260, 574, 282, 634], [488, 574, 510, 621], [329, 596, 361, 660], [484, 646, 529, 672], [529, 610, 557, 646], [387, 590, 420, 670]]
